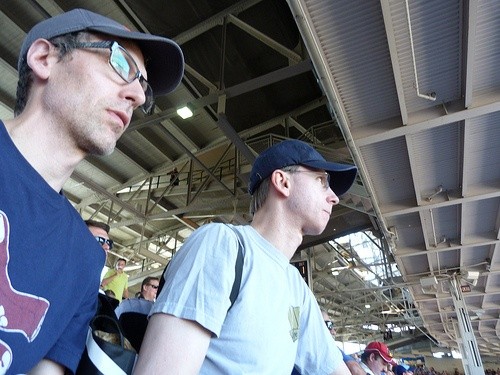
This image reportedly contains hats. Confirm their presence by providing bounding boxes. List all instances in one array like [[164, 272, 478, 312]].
[[248, 140, 358, 196], [395, 364, 413, 373], [18, 9, 184, 96], [365, 342, 392, 362]]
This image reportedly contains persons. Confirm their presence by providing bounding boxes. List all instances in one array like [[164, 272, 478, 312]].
[[101, 258, 130, 301], [321, 308, 367, 375], [132, 138, 357, 375], [359, 341, 396, 375], [382, 359, 415, 375], [0, 8, 185, 375], [139, 277, 160, 302], [84, 219, 112, 256]]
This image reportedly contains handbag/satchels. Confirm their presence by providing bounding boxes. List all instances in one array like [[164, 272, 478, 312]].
[[75, 315, 139, 375]]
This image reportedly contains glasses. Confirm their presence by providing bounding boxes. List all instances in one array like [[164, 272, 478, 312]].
[[284, 170, 330, 191], [52, 40, 153, 114], [95, 236, 113, 249], [148, 284, 159, 289], [325, 321, 333, 329]]
